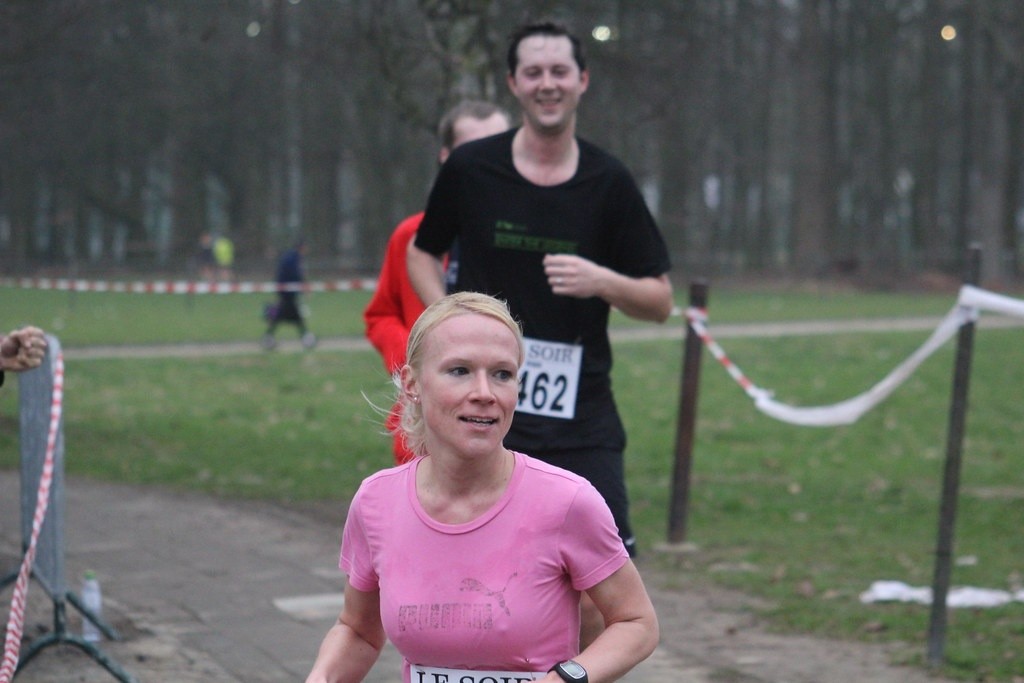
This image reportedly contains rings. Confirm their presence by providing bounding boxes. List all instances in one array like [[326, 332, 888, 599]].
[[557, 277, 563, 286]]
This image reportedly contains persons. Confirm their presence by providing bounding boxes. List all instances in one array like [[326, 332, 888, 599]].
[[361, 97, 510, 467], [305, 291, 659, 683], [262, 240, 321, 351], [406, 23, 673, 655], [0, 327, 48, 385], [196, 229, 234, 281]]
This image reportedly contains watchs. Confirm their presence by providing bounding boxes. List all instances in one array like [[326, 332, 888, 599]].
[[547, 659, 589, 683]]
